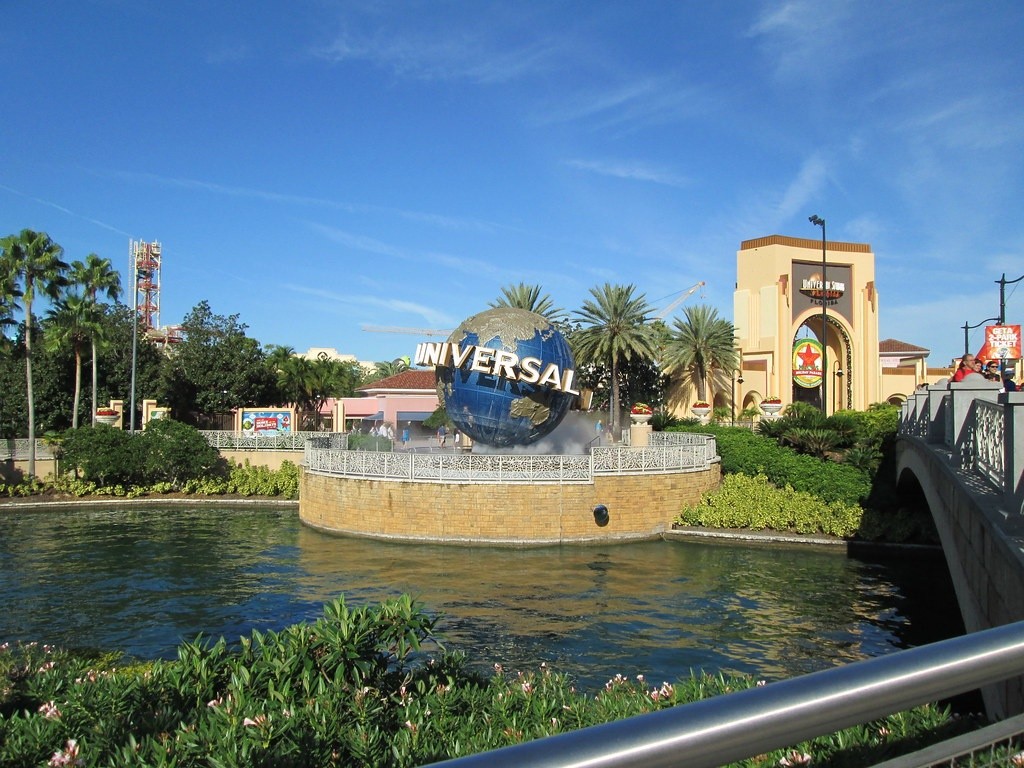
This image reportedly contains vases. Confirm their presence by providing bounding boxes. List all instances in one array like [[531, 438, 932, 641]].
[[759, 404, 783, 415], [630, 414, 652, 425], [95, 415, 120, 425], [691, 408, 712, 419]]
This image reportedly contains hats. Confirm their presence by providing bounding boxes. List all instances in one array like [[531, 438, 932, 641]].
[[1005, 368, 1015, 374]]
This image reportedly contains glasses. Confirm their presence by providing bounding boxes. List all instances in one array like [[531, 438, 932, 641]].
[[989, 365, 998, 368]]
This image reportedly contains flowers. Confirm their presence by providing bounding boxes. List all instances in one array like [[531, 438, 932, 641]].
[[761, 395, 781, 404], [693, 400, 710, 408], [97, 408, 119, 415], [631, 402, 652, 414]]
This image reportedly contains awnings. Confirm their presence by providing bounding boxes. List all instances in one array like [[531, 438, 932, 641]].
[[362, 410, 384, 421], [397, 412, 449, 422]]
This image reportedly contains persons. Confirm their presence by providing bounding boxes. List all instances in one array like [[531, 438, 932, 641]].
[[569, 399, 609, 413], [948, 353, 1024, 393], [917, 383, 930, 391], [436, 423, 460, 449], [351, 421, 396, 448], [402, 421, 411, 452], [595, 419, 613, 443], [814, 396, 821, 409]]
